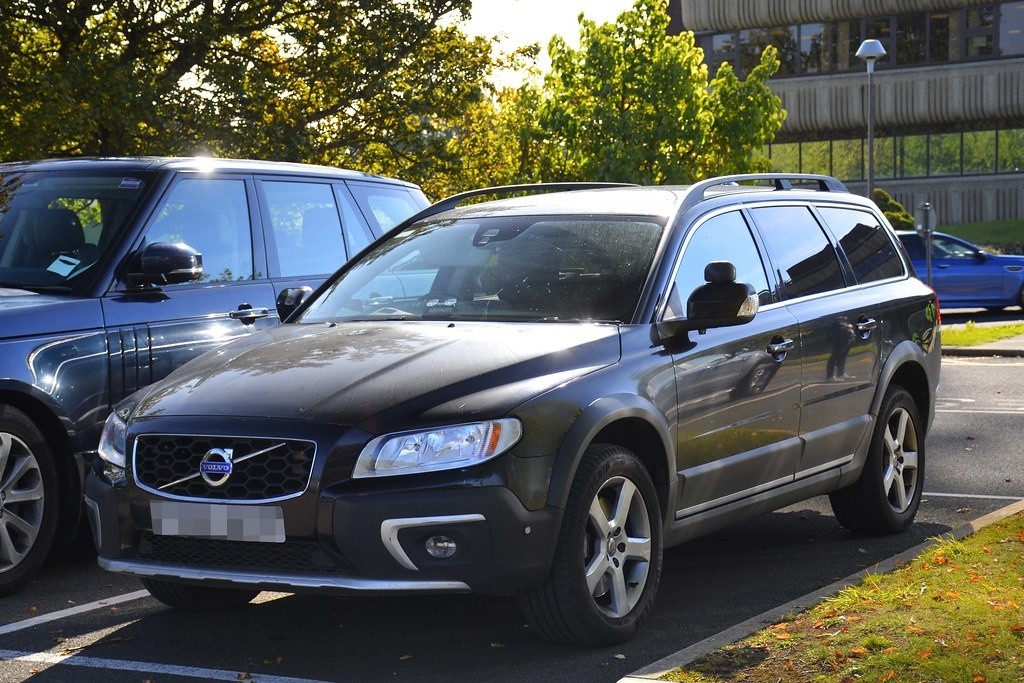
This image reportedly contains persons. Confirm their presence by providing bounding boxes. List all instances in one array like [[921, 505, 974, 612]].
[[826, 317, 856, 382]]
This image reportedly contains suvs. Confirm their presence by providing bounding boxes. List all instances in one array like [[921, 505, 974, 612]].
[[84, 175, 942, 648]]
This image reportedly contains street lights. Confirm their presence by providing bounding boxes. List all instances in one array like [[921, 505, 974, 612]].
[[855, 39, 888, 201]]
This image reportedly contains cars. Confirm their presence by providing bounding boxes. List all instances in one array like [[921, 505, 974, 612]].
[[0, 156, 444, 593], [895, 229, 1024, 311]]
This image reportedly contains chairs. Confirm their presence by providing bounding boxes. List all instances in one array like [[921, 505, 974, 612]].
[[30, 207, 97, 272], [461, 258, 549, 314], [182, 209, 235, 281]]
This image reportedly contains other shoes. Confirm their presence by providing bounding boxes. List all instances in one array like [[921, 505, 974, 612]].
[[837, 373, 856, 380], [825, 376, 844, 382]]
